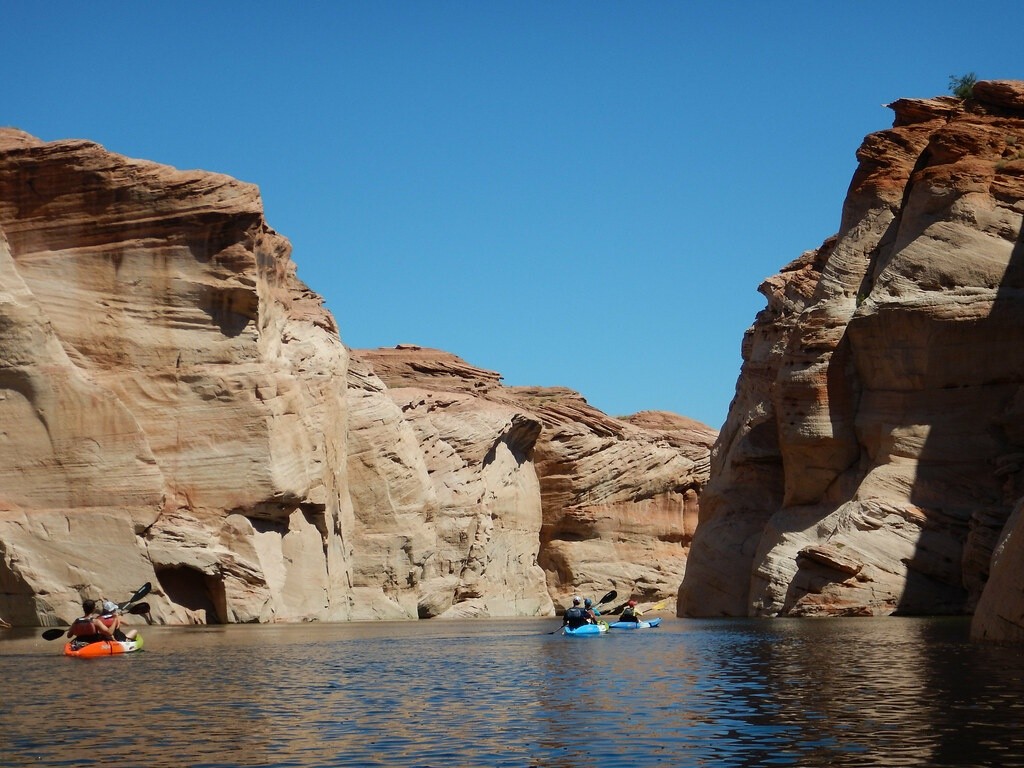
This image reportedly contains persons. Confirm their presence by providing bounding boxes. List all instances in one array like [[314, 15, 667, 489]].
[[562, 597, 605, 631], [67, 599, 137, 644], [618, 600, 644, 623]]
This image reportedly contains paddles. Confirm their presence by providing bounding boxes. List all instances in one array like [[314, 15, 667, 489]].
[[42, 581, 152, 641], [548, 590, 618, 635], [635, 600, 668, 617]]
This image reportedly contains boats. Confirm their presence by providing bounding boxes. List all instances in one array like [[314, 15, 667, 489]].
[[563, 620, 609, 633], [608, 618, 664, 630], [64, 634, 142, 658]]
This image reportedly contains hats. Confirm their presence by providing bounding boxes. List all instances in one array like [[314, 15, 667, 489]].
[[573, 597, 581, 603], [629, 601, 634, 607], [104, 602, 119, 612], [585, 599, 592, 606]]
